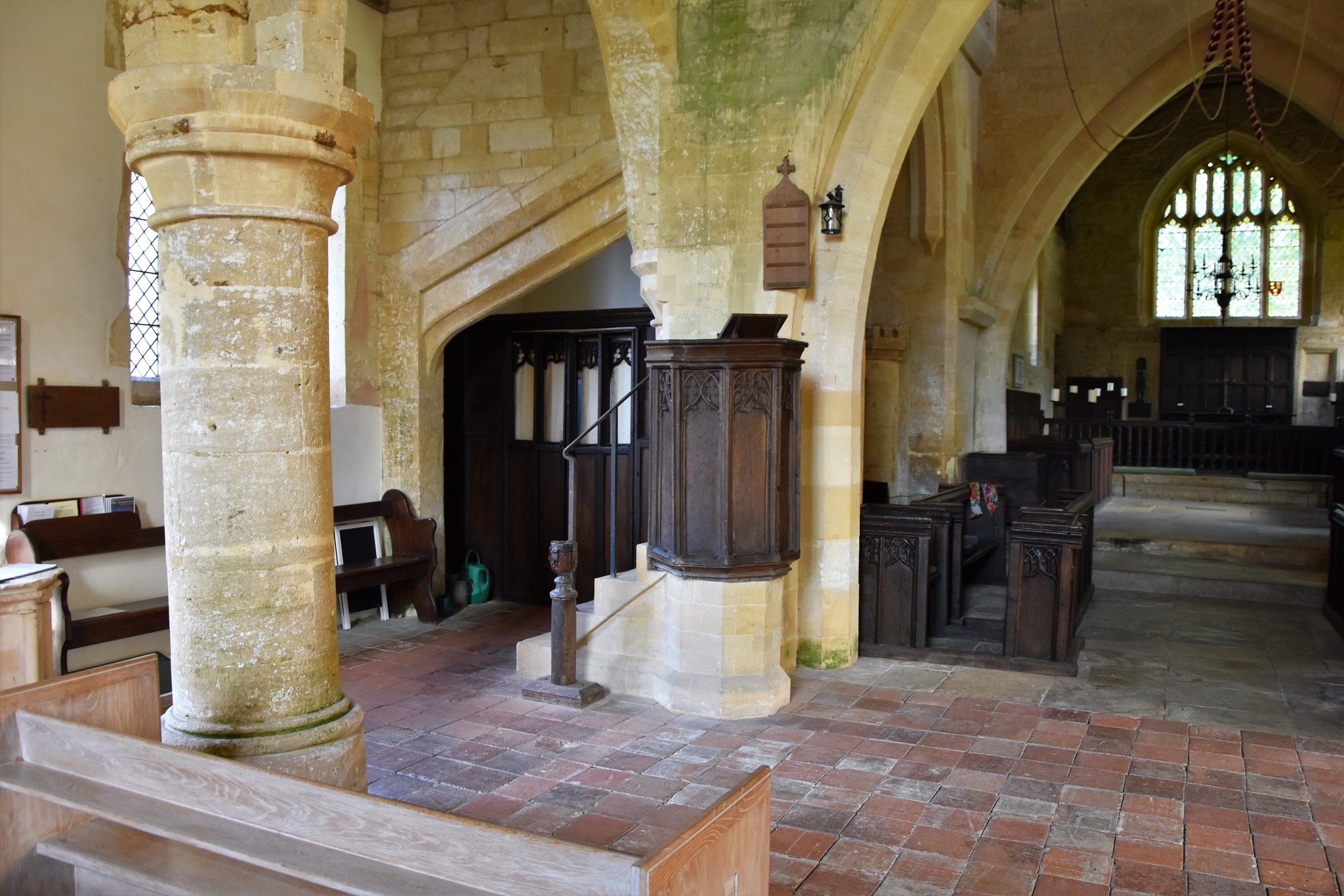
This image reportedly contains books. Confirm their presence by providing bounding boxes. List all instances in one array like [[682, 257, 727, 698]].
[[17, 496, 136, 526]]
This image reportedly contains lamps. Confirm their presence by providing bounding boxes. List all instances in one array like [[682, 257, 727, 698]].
[[818, 185, 846, 235], [1185, 82, 1264, 327]]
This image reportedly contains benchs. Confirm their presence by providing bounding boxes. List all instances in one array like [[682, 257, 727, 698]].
[[9, 487, 440, 674], [912, 482, 1008, 581]]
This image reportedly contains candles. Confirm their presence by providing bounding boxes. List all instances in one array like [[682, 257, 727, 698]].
[[1069, 386, 1079, 393], [1120, 388, 1128, 397], [1051, 388, 1061, 402], [1088, 390, 1097, 403], [1107, 382, 1114, 391], [1329, 393, 1337, 402], [1095, 388, 1101, 397]]
